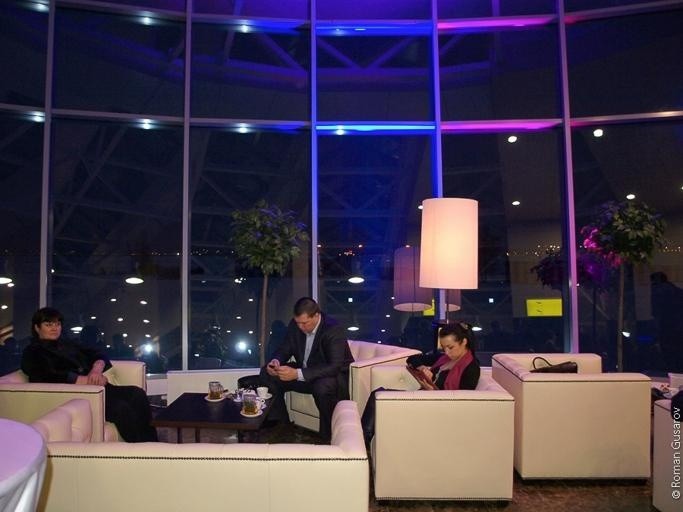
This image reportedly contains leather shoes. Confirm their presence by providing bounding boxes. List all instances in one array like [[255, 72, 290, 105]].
[[264, 428, 280, 444]]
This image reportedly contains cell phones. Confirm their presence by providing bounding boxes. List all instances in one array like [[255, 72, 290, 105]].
[[269, 365, 278, 371]]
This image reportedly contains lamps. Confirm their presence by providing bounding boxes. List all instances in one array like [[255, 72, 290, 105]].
[[418, 196, 482, 332], [444, 287, 461, 312], [391, 246, 432, 313]]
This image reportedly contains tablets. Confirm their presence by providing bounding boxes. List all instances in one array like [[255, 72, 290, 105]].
[[405, 365, 438, 390]]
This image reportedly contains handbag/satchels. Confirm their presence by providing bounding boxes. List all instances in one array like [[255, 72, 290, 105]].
[[528, 357, 576, 373]]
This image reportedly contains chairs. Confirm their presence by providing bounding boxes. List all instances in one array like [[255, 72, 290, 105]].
[[492, 350, 654, 485], [371, 363, 514, 504], [648, 382, 683, 511], [273, 339, 424, 435], [1, 354, 149, 443]]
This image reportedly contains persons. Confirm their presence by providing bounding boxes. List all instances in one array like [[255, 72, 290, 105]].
[[257, 297, 356, 446], [20, 306, 159, 443], [360, 321, 481, 451]]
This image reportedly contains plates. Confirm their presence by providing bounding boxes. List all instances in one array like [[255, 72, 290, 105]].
[[240, 409, 262, 417], [205, 395, 224, 402], [256, 393, 272, 399]]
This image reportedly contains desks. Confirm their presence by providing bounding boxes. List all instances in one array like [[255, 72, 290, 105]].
[[0, 419, 50, 512]]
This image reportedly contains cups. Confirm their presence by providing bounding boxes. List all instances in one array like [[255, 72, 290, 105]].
[[243, 394, 256, 413], [242, 389, 255, 395], [255, 386, 268, 397], [207, 380, 222, 399], [234, 391, 243, 400]]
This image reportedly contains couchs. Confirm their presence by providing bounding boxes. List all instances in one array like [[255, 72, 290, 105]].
[[24, 398, 371, 512]]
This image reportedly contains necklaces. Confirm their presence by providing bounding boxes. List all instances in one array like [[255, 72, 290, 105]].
[[433, 360, 454, 384]]
